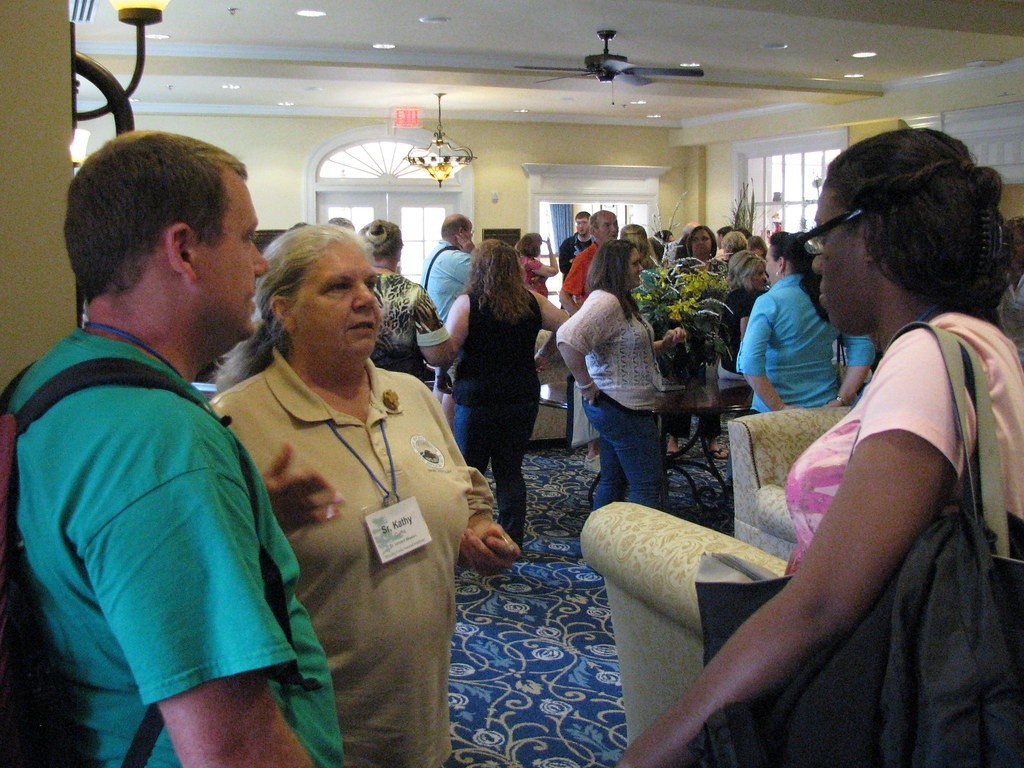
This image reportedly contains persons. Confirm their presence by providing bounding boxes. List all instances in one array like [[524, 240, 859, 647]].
[[329, 218, 452, 380], [420, 213, 476, 432], [521, 232, 559, 299], [435, 240, 569, 550], [211, 225, 520, 768], [559, 209, 878, 514], [0, 131, 345, 768], [619, 129, 1024, 768]]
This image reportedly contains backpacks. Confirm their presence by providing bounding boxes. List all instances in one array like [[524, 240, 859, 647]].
[[1, 360, 320, 768]]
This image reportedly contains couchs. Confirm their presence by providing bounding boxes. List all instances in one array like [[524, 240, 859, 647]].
[[728, 407, 851, 560], [580, 500, 785, 768], [530, 330, 568, 440]]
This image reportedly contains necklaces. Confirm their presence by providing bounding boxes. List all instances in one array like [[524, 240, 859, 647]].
[[84, 321, 184, 378]]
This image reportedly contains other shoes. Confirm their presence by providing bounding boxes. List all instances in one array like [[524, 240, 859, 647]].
[[584, 456, 603, 473]]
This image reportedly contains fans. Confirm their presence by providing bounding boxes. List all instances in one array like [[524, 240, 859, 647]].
[[514, 30, 704, 84]]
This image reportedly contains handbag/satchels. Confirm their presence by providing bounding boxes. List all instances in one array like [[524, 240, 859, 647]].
[[695, 323, 1023, 768]]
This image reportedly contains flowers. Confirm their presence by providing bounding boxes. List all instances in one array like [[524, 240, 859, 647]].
[[626, 256, 742, 360]]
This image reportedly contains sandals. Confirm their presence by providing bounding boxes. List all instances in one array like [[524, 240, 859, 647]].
[[710, 449, 728, 460]]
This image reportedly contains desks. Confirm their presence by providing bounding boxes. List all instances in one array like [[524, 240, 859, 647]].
[[588, 379, 760, 516]]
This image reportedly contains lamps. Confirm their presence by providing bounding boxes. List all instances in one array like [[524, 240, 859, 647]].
[[405, 93, 477, 187], [71, 0, 172, 175]]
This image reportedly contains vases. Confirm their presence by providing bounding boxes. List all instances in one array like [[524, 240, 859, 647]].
[[662, 327, 707, 378]]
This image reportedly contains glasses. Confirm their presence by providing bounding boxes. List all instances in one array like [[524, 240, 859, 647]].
[[795, 205, 863, 255], [740, 255, 759, 270], [466, 229, 474, 234]]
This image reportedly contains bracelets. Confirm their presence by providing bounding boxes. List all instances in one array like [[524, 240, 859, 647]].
[[538, 350, 552, 360], [836, 396, 847, 405]]
